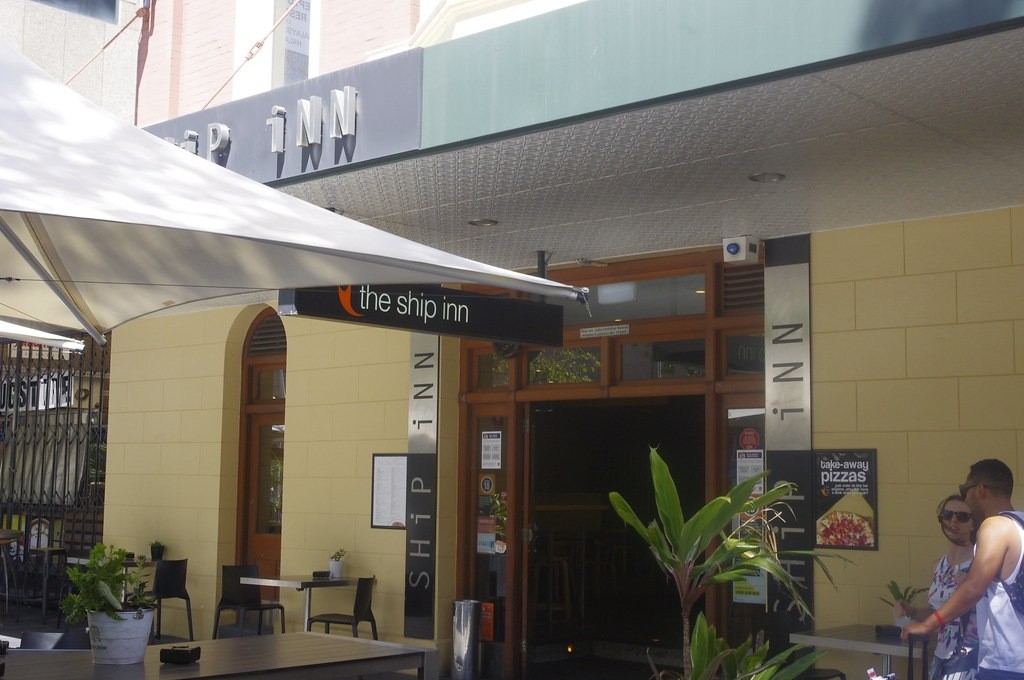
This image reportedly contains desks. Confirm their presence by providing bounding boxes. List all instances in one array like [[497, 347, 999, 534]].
[[89, 559, 156, 603], [529, 503, 610, 641], [0, 630, 440, 680], [0, 529, 24, 621], [240, 576, 359, 632], [789, 624, 938, 680]]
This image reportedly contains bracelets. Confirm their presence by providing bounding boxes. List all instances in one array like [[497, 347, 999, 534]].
[[934, 610, 945, 626]]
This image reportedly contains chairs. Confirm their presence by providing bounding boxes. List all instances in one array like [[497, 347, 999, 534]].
[[764, 607, 846, 679], [124, 558, 194, 645], [307, 574, 378, 640], [212, 565, 286, 639], [13, 631, 64, 649], [56, 551, 88, 628]]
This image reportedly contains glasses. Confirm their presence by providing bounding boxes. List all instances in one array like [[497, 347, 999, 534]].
[[940, 510, 973, 523], [959, 484, 989, 501]]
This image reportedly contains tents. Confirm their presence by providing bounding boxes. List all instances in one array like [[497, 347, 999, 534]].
[[0, 30, 589, 349], [0, 320, 85, 353]]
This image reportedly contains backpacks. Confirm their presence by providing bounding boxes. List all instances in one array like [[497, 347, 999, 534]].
[[993, 512, 1024, 614]]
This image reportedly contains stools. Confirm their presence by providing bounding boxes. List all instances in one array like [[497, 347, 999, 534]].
[[17, 547, 68, 626], [529, 523, 631, 633]]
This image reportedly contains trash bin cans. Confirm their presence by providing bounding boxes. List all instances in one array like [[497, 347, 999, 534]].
[[452, 600, 483, 680]]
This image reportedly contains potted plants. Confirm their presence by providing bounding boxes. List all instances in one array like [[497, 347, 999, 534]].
[[147, 540, 164, 561], [879, 580, 931, 626], [329, 549, 346, 578], [59, 543, 158, 663]]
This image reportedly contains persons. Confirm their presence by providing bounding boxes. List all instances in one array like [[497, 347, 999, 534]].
[[893, 457, 1024, 680]]
